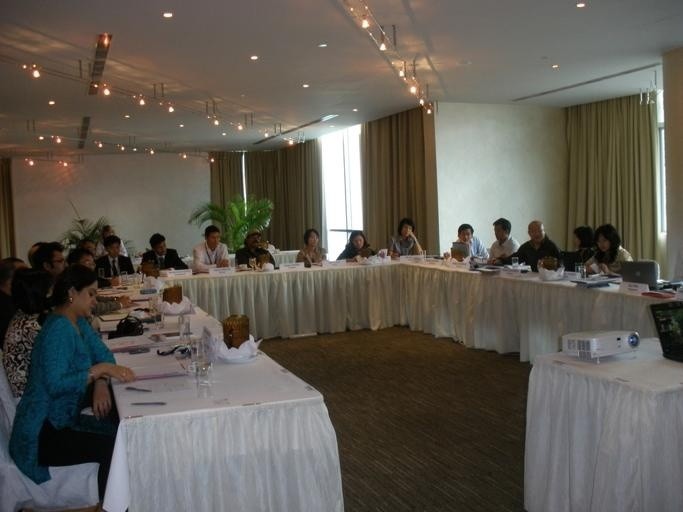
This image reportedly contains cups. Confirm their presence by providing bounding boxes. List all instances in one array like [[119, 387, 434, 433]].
[[99, 264, 250, 381]]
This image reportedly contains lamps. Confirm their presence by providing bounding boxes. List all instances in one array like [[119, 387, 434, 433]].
[[341, 0, 435, 117]]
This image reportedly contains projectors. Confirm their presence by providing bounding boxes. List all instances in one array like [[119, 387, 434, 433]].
[[561, 328, 641, 360]]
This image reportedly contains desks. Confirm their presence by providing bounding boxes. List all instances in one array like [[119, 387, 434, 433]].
[[88, 251, 682, 512]]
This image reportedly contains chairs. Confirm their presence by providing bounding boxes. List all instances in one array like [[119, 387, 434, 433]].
[[0, 351, 101, 511]]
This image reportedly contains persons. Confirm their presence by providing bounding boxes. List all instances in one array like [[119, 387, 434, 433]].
[[3, 226, 134, 507], [335, 232, 375, 259], [141, 235, 192, 271], [297, 229, 324, 261], [457, 219, 636, 279], [390, 220, 422, 259], [235, 229, 276, 268], [191, 224, 230, 275]]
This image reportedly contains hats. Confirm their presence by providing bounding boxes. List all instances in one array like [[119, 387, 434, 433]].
[[244, 225, 261, 244]]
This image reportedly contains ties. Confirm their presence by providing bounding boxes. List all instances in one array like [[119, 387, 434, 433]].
[[112, 259, 118, 277], [159, 256, 165, 268]]
